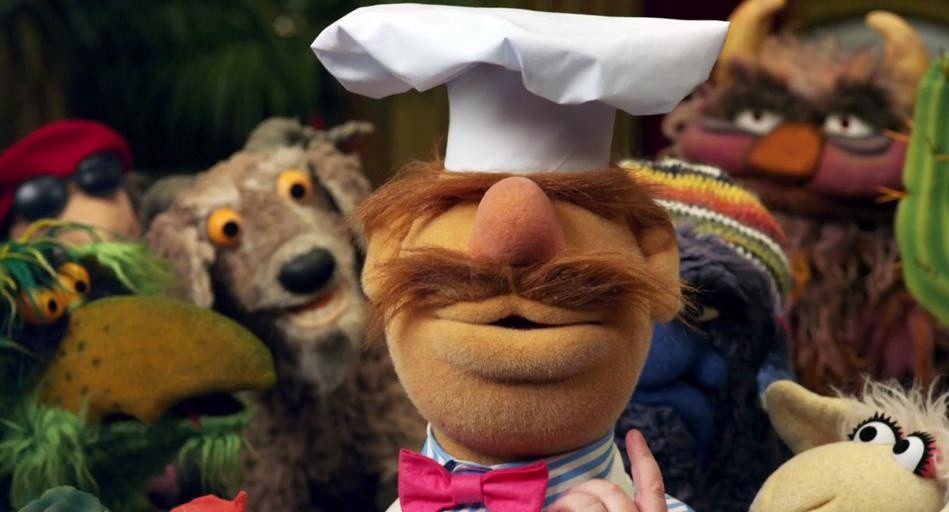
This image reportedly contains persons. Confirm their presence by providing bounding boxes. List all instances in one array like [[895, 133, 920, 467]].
[[545, 427, 668, 510]]
